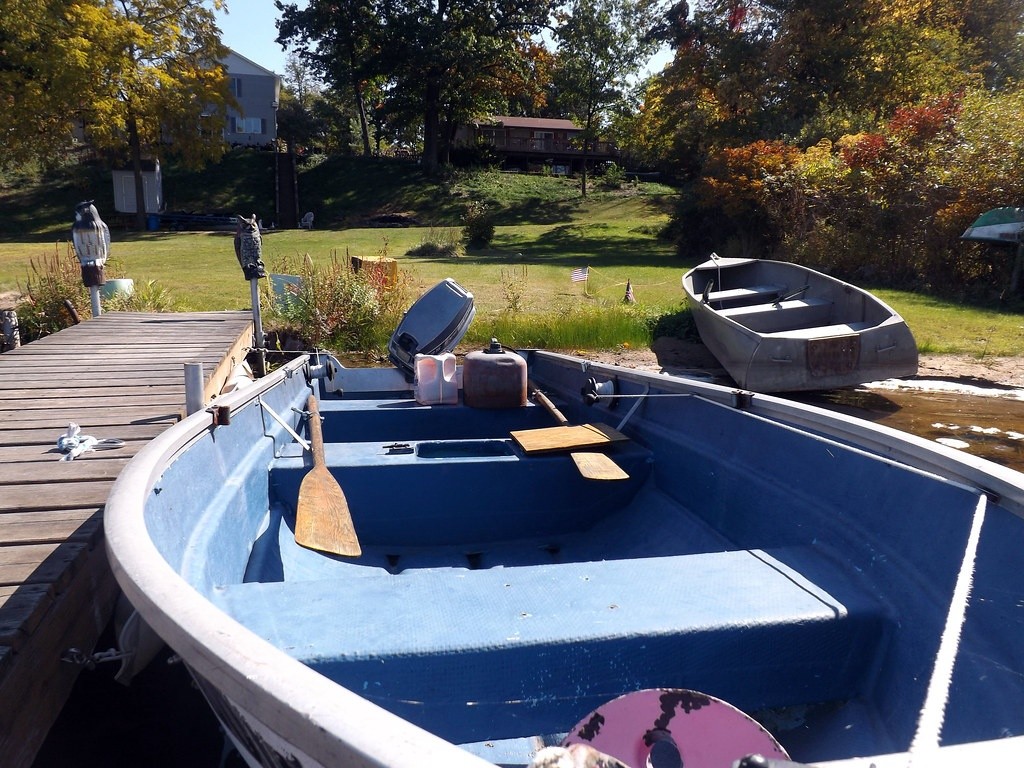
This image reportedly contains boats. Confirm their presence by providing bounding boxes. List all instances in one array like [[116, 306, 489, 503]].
[[102, 279, 1023, 768], [682, 252, 918, 394]]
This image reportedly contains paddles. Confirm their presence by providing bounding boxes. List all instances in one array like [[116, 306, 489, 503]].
[[294, 392, 363, 559]]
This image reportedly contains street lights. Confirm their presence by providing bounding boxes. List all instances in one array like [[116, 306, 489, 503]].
[[272, 100, 280, 228]]
